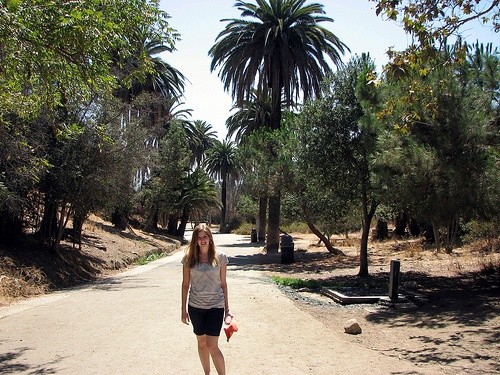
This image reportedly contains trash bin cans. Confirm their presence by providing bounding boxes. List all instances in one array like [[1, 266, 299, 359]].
[[251, 228, 257, 242], [280, 235, 294, 264]]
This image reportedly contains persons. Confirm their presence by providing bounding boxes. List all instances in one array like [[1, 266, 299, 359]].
[[181, 225, 233, 375]]
